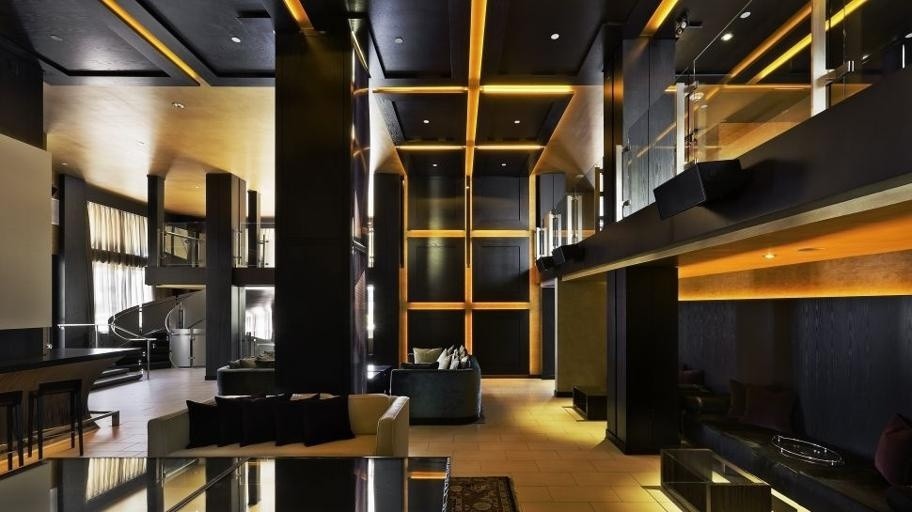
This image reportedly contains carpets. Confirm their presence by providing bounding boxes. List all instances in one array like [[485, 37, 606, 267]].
[[448, 473, 521, 512]]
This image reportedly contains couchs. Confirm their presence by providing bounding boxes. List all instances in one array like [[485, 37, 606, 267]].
[[147, 389, 412, 456], [392, 352, 484, 425], [681, 393, 911, 512], [217, 363, 274, 395]]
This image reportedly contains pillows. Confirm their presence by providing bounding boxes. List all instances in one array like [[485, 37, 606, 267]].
[[228, 358, 276, 369], [872, 413, 911, 486], [412, 344, 472, 369], [724, 375, 783, 420], [679, 366, 704, 384], [737, 387, 796, 436], [184, 397, 219, 449], [214, 393, 268, 447], [238, 392, 294, 446], [268, 392, 322, 446], [301, 389, 357, 446]]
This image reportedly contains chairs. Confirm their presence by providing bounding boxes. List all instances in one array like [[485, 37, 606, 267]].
[[28, 376, 85, 460], [0, 389, 25, 471]]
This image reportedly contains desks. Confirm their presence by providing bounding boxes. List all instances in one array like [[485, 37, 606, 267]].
[[1, 345, 144, 456], [0, 455, 452, 511], [572, 384, 608, 421], [659, 447, 773, 510]]
